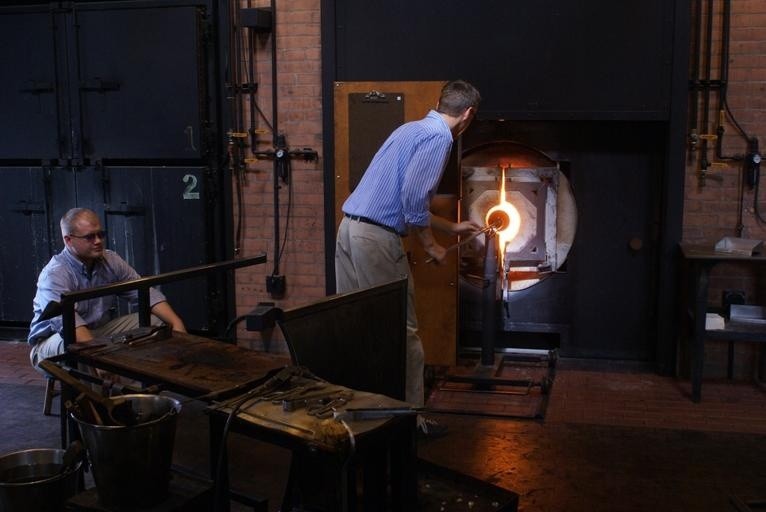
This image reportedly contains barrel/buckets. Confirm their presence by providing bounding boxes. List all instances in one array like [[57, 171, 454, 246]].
[[69, 392, 183, 504], [0, 447, 86, 512]]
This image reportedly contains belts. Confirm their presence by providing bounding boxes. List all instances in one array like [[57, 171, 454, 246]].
[[344, 213, 399, 235]]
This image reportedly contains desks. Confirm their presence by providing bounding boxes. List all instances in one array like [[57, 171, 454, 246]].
[[69, 326, 427, 510]]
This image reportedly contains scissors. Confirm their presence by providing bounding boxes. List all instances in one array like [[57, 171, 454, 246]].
[[308, 395, 355, 419], [260, 381, 328, 404]]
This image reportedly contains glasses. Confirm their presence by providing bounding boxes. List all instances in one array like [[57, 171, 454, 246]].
[[70, 231, 105, 242]]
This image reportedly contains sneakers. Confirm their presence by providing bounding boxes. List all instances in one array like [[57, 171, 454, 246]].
[[417, 416, 449, 439]]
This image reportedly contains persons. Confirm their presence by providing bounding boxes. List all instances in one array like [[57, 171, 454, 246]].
[[28, 208, 187, 394], [335, 79, 482, 439]]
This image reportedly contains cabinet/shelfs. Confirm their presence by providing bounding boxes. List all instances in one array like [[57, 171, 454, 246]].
[[676, 239, 764, 405]]
